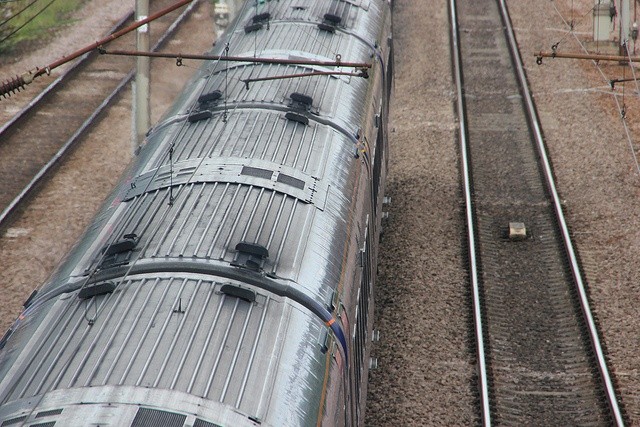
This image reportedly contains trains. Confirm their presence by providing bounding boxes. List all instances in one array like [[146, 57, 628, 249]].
[[1, 0, 395, 426]]
